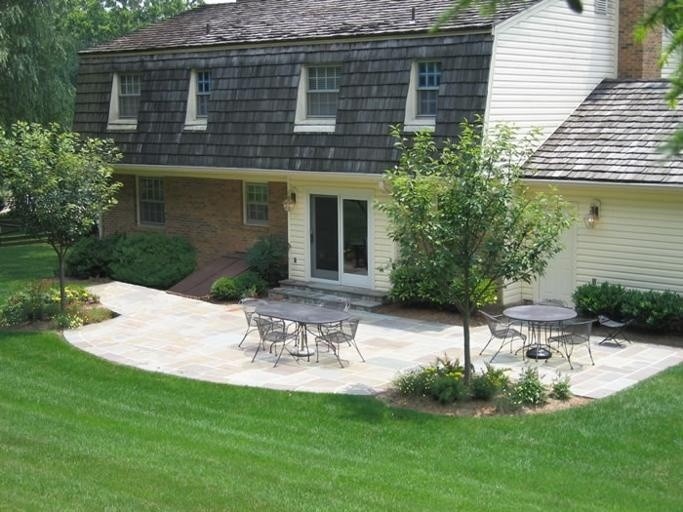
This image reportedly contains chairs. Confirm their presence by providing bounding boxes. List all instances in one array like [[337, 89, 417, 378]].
[[544, 319, 597, 370], [528, 298, 568, 348], [315, 318, 365, 368], [252, 316, 299, 368], [305, 297, 350, 351], [239, 297, 285, 351], [477, 309, 527, 363]]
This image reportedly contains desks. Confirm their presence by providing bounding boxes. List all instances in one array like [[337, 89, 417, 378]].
[[502, 304, 578, 360], [256, 302, 350, 357]]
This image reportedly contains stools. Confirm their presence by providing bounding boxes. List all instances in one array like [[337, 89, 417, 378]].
[[598, 314, 635, 347]]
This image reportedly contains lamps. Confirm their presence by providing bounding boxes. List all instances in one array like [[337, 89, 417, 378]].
[[584, 199, 601, 231], [282, 186, 296, 212]]
